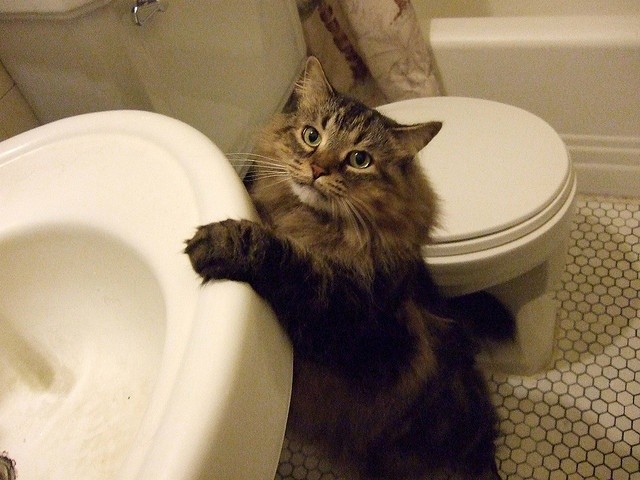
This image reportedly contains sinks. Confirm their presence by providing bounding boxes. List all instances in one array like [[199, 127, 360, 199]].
[[0, 110, 295, 479]]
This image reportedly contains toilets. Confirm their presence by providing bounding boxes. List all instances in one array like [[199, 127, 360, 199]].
[[0, 2, 576, 376]]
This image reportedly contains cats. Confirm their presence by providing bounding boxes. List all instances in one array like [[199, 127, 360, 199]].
[[182, 55, 522, 477]]
